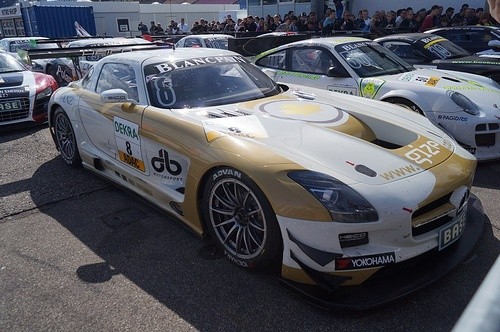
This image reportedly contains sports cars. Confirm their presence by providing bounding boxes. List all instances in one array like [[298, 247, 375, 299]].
[[46, 46, 479, 289]]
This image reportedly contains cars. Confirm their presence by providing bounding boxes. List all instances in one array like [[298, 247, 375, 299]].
[[225, 35, 500, 162], [373, 25, 500, 83], [0, 50, 59, 132], [0, 31, 314, 88]]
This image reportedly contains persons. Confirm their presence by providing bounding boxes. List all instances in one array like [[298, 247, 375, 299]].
[[138, 4, 500, 43]]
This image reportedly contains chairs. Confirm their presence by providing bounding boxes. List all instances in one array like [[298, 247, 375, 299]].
[[170, 66, 226, 101]]
